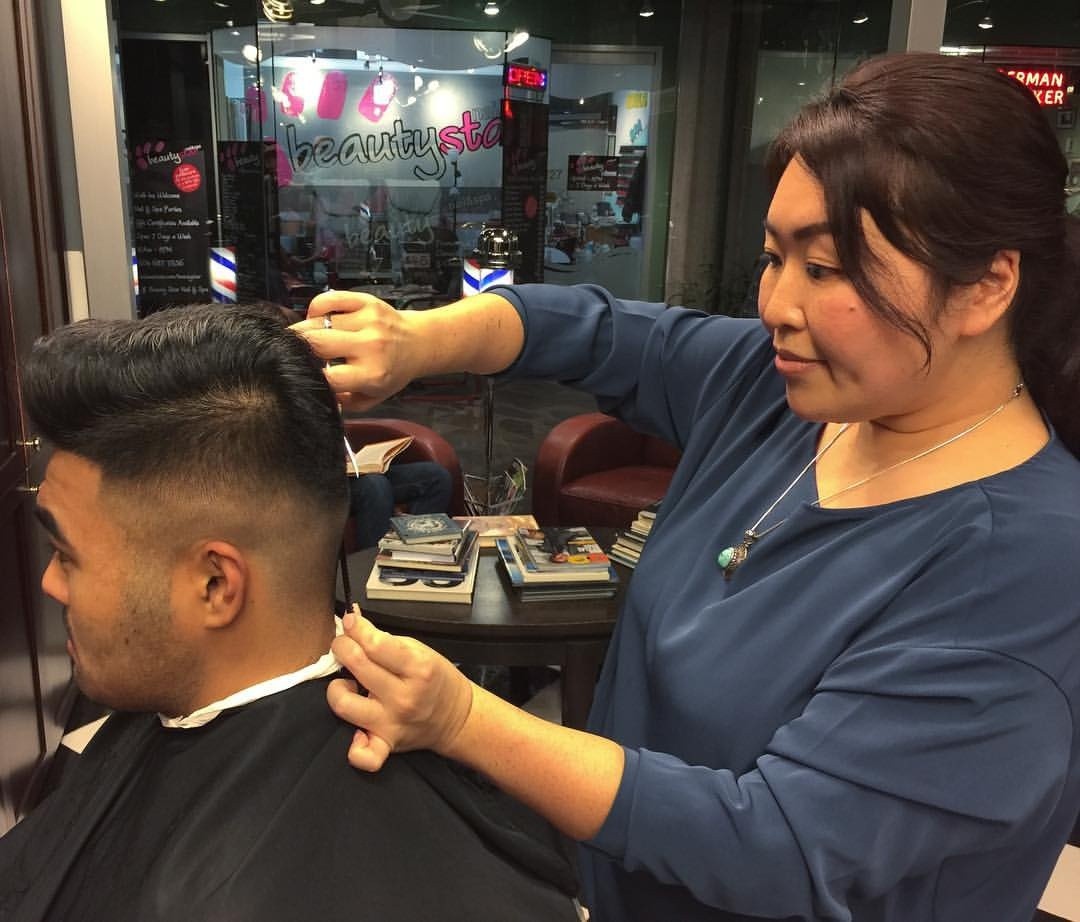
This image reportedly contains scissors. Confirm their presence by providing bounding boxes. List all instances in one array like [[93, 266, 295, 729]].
[[323, 284, 360, 479]]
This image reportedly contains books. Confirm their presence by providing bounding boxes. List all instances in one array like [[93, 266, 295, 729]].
[[363, 507, 659, 604], [345, 436, 412, 475]]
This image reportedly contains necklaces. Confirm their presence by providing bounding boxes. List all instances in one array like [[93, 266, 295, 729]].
[[716, 381, 1025, 578]]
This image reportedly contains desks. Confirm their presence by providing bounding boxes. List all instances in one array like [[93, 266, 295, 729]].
[[333, 546, 634, 731]]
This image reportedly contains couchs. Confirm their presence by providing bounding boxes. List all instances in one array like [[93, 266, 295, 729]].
[[338, 419, 465, 554], [533, 411, 684, 529]]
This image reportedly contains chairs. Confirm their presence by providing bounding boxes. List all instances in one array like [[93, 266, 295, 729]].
[[400, 292, 485, 399]]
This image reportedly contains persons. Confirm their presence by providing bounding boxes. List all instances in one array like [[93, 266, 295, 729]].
[[621, 149, 647, 232], [0, 302, 590, 922], [286, 51, 1080, 922], [345, 460, 453, 551]]
